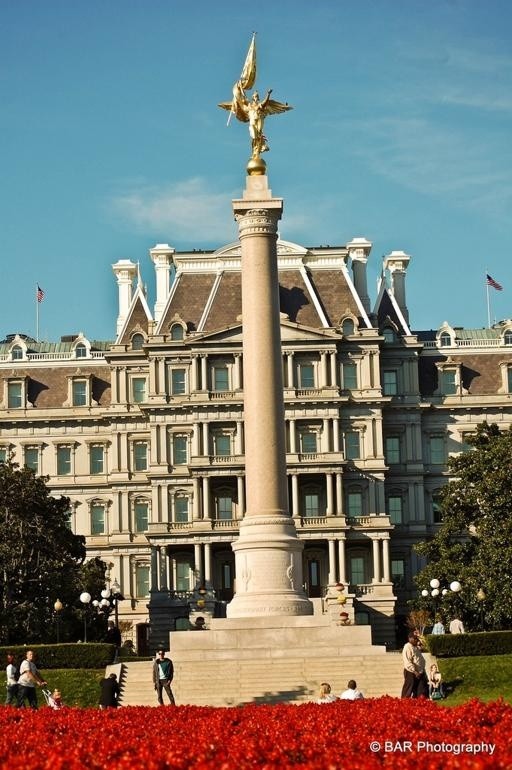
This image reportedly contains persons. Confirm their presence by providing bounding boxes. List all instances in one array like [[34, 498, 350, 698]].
[[102, 621, 121, 656], [428, 664, 448, 701], [152, 649, 175, 705], [15, 650, 47, 710], [52, 688, 67, 708], [340, 679, 363, 700], [450, 614, 466, 634], [316, 682, 339, 704], [432, 617, 447, 634], [235, 88, 273, 157], [4, 655, 26, 708], [400, 632, 426, 698], [416, 639, 430, 698], [96, 672, 120, 710]]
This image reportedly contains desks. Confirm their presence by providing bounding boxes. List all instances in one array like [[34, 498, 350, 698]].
[[80, 590, 110, 643], [422, 579, 461, 627], [54, 599, 62, 643], [477, 588, 486, 631]]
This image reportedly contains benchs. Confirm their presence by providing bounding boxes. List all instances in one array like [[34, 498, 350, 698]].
[[230, 32, 257, 115], [36, 286, 46, 303], [486, 273, 505, 292]]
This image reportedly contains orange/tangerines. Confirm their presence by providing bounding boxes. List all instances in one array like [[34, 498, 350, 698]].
[[41, 686, 68, 710]]
